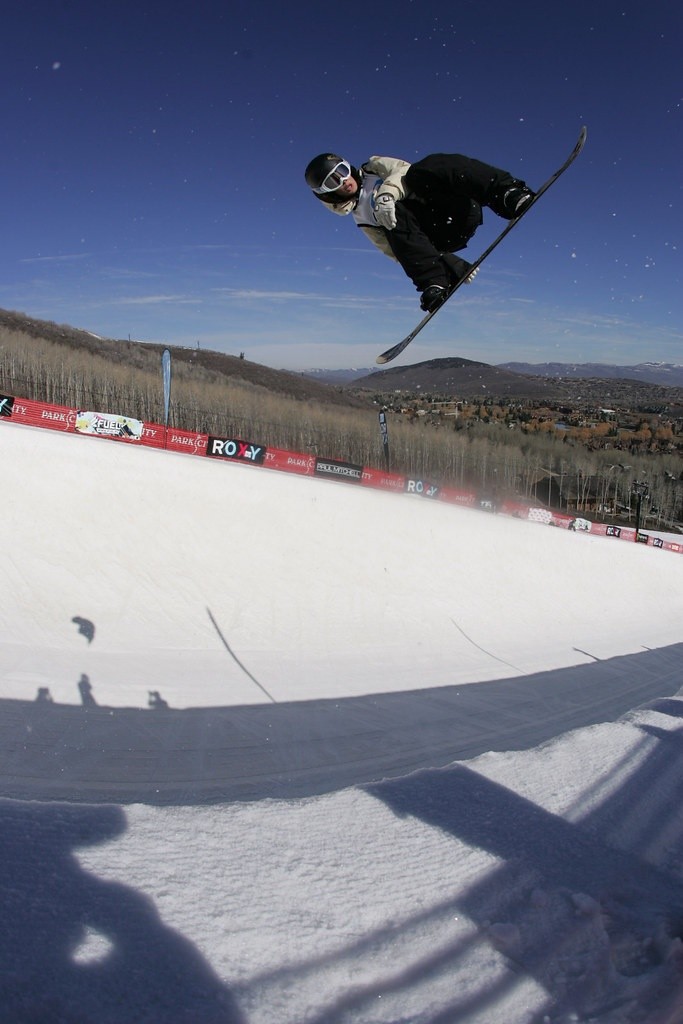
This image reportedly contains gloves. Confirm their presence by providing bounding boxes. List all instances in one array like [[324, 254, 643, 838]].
[[374, 193, 397, 230]]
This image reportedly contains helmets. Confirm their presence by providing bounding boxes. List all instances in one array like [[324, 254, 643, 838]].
[[305, 153, 362, 203]]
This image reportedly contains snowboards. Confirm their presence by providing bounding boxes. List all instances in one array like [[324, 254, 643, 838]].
[[373, 125, 593, 367]]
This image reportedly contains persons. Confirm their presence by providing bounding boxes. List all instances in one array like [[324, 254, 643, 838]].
[[302, 145, 537, 315]]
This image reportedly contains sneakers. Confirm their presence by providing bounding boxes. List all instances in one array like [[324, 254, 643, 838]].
[[503, 185, 536, 216], [420, 274, 457, 313]]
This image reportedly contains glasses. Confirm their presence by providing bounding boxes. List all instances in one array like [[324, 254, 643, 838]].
[[311, 158, 350, 194]]
